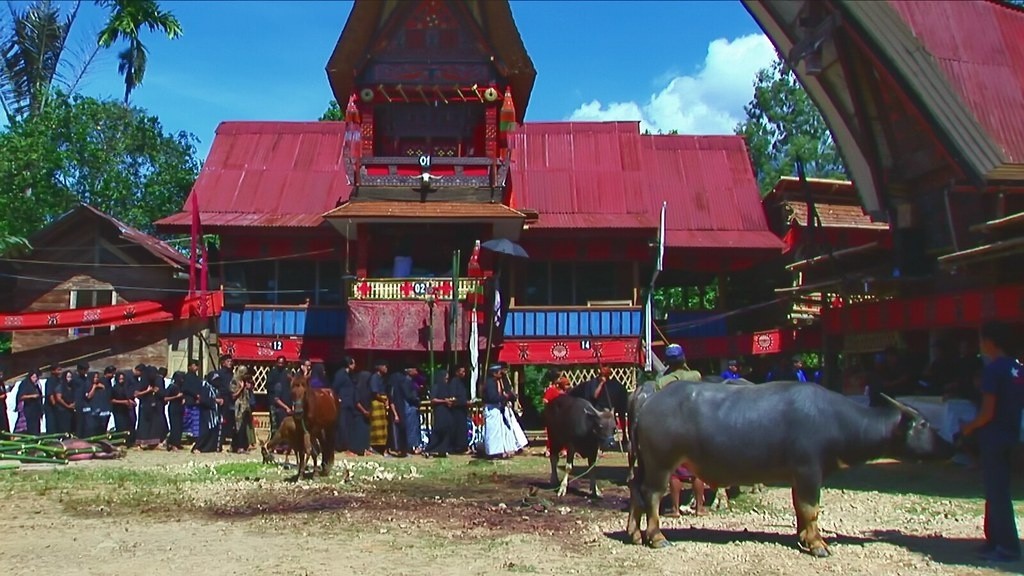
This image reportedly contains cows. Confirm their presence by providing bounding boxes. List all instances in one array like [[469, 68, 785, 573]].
[[545, 395, 619, 498], [627, 381, 957, 557], [410, 172, 444, 183]]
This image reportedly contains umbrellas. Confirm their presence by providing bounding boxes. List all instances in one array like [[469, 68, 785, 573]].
[[481, 238, 530, 276]]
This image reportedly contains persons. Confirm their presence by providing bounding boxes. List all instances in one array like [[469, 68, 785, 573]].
[[12, 354, 256, 453], [0, 372, 10, 433], [267, 355, 528, 459], [543, 343, 842, 517], [867, 323, 1024, 559]]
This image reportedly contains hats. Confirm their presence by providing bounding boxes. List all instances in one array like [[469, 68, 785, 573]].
[[664, 343, 683, 360]]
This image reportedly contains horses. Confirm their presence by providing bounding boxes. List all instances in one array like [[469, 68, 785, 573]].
[[257, 370, 340, 481]]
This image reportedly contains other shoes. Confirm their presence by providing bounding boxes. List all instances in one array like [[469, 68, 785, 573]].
[[974, 543, 1021, 561]]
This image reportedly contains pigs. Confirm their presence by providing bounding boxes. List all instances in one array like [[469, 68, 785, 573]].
[[20, 439, 127, 463]]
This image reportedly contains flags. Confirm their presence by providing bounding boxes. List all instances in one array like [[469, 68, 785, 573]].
[[752, 329, 781, 354]]
